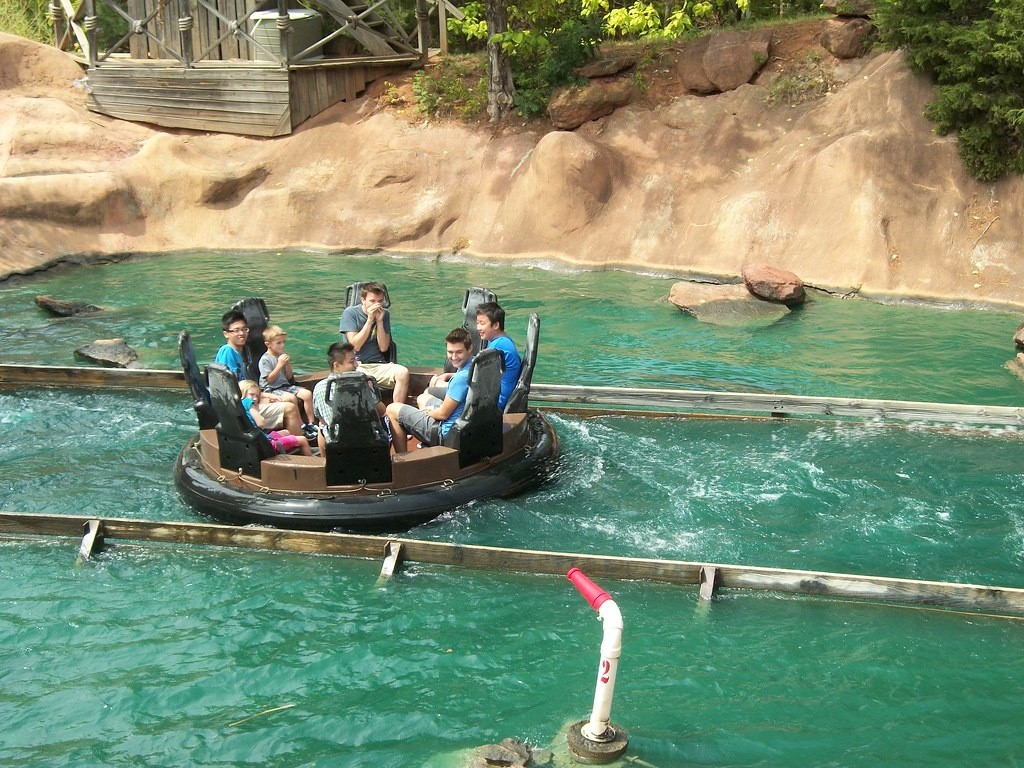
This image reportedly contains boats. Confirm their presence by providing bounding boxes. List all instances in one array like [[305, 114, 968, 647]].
[[174, 368, 558, 537]]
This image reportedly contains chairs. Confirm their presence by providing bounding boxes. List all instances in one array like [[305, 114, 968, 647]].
[[202, 364, 300, 481], [505, 314, 541, 413], [176, 328, 220, 430], [231, 297, 271, 382], [322, 374, 392, 487], [418, 348, 504, 470], [443, 287, 499, 375], [342, 282, 397, 364]]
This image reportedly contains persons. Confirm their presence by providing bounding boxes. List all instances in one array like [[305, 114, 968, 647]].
[[386, 328, 475, 455], [214, 311, 326, 457], [257, 325, 322, 442], [423, 302, 523, 416], [314, 342, 392, 458], [235, 380, 314, 457], [338, 281, 414, 441]]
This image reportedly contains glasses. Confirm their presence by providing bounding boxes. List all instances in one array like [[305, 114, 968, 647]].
[[222, 327, 250, 332]]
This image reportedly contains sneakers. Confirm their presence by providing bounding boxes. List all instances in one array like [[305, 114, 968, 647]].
[[309, 422, 320, 431], [300, 424, 318, 442]]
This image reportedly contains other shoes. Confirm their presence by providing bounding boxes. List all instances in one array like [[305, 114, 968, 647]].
[[417, 441, 429, 448]]
